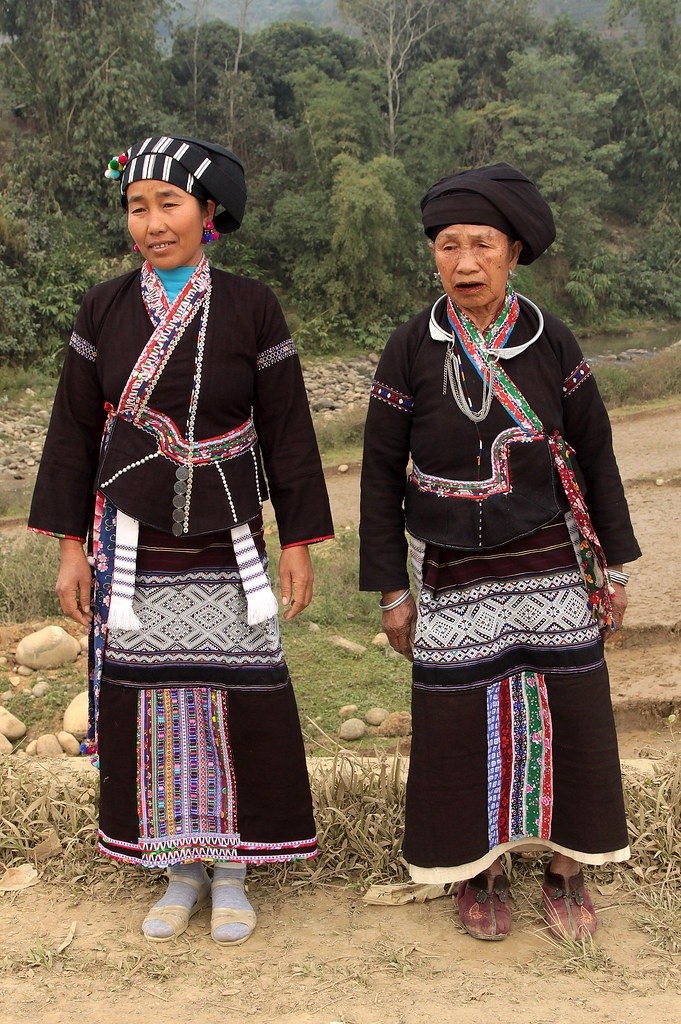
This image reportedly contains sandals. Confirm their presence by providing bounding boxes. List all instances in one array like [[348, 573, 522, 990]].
[[142, 876, 210, 942], [208, 879, 257, 946]]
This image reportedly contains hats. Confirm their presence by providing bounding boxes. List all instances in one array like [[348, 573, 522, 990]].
[[103, 135, 247, 235], [420, 163, 555, 266]]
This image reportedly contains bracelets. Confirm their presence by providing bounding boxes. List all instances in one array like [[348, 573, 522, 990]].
[[608, 569, 630, 586], [379, 589, 411, 610]]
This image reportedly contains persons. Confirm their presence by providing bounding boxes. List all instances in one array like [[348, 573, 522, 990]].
[[27, 136, 336, 947], [357, 165, 641, 945]]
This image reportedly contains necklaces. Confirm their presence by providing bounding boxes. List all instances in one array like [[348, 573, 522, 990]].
[[428, 289, 545, 422]]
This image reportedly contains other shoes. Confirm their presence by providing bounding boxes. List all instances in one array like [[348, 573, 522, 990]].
[[456, 873, 512, 939], [542, 861, 597, 943]]
